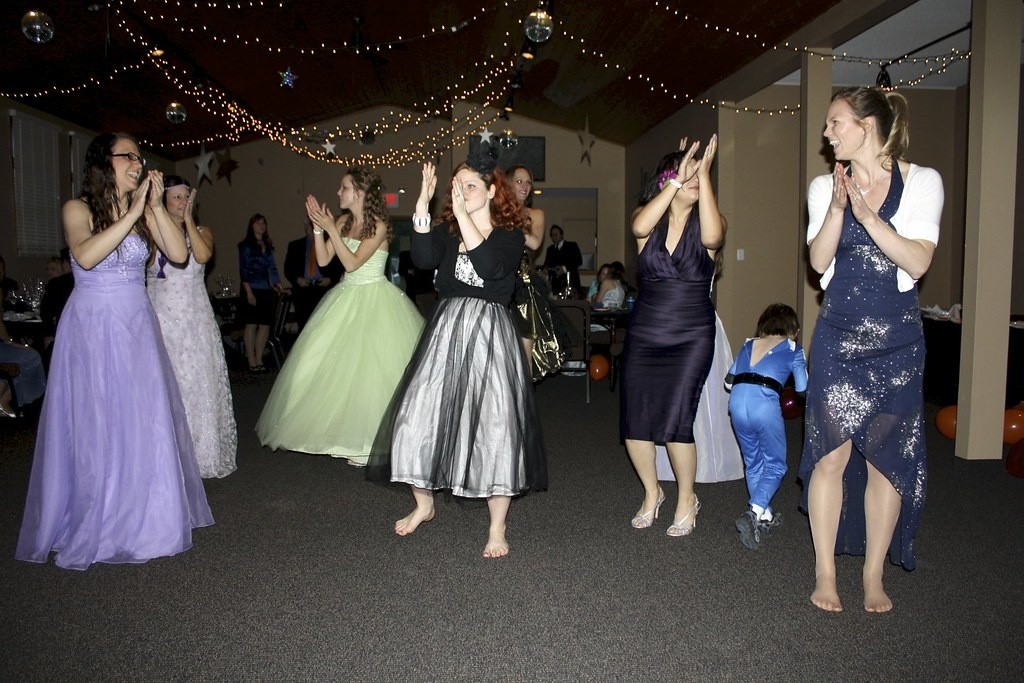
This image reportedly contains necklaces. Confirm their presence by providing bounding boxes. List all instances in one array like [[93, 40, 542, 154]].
[[113, 201, 128, 221], [853, 170, 884, 195]]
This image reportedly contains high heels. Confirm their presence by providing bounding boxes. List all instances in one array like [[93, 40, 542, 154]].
[[631, 484, 666, 529], [667, 493, 701, 536]]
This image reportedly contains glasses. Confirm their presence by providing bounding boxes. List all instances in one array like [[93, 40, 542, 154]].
[[111, 152, 147, 167]]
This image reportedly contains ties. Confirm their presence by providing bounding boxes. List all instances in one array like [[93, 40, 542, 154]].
[[309, 239, 317, 277], [556, 244, 558, 249]]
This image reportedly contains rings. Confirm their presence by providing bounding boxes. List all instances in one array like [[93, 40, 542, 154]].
[[188, 201, 192, 203]]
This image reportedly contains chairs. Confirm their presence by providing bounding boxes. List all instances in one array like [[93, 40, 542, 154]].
[[236, 287, 294, 371], [553, 299, 591, 404]]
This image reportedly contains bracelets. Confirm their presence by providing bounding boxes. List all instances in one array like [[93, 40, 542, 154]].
[[4, 336, 13, 344], [412, 213, 431, 226], [670, 179, 682, 188], [313, 229, 324, 235]]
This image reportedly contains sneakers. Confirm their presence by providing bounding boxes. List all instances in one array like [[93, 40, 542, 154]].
[[757, 511, 784, 539], [735, 506, 760, 550]]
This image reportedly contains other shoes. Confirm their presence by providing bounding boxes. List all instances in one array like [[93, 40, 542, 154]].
[[0, 406, 24, 418]]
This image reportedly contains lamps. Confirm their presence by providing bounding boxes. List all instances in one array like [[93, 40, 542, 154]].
[[165, 101, 187, 125], [20, 10, 54, 43]]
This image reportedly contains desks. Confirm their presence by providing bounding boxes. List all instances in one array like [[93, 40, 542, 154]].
[[588, 309, 628, 391], [3, 320, 57, 349]]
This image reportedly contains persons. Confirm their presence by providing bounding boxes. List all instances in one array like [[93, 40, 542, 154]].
[[620, 133, 728, 537], [284, 215, 344, 330], [587, 262, 626, 309], [798, 86, 944, 611], [237, 213, 284, 372], [390, 162, 547, 559], [497, 160, 545, 373], [546, 224, 583, 298], [0, 247, 75, 417], [724, 302, 809, 550], [15, 137, 214, 571], [147, 178, 237, 479], [254, 166, 426, 466]]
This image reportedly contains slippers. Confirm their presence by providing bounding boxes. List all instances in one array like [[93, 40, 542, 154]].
[[250, 364, 265, 371]]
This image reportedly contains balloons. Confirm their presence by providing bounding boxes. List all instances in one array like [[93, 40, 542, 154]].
[[590, 355, 609, 380]]
